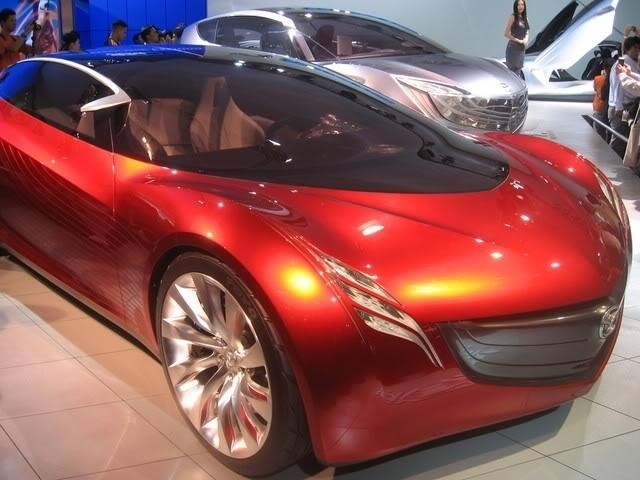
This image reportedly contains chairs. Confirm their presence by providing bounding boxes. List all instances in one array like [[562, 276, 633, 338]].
[[587, 50, 615, 79], [189, 75, 265, 152], [128, 99, 194, 156], [312, 24, 337, 59]]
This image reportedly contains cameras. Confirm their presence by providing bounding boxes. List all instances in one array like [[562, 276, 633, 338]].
[[31, 20, 41, 32]]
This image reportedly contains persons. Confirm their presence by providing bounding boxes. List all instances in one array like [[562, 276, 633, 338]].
[[504, 0, 530, 76], [1, 7, 184, 73], [592, 25, 640, 167]]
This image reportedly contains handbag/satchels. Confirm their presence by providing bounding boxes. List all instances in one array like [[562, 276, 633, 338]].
[[622, 104, 640, 168]]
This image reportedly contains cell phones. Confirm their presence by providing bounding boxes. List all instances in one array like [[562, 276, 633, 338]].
[[618, 57, 624, 65]]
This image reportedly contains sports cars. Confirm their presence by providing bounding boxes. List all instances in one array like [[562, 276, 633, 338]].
[[1, 47, 634, 479], [473, 0, 640, 104], [179, 7, 531, 146]]
